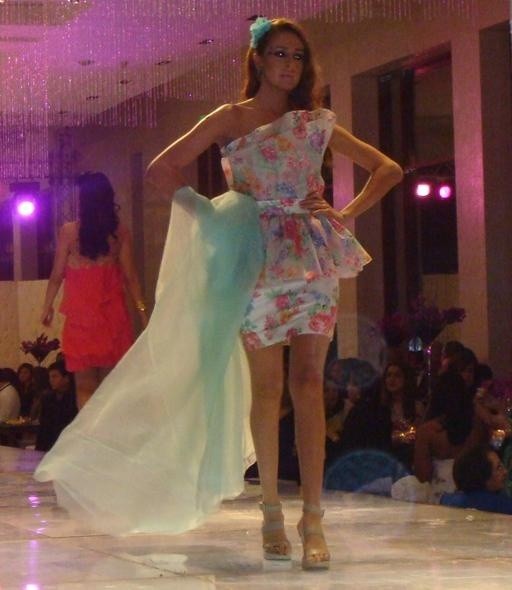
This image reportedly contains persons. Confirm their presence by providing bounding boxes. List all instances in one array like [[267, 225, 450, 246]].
[[1, 352, 80, 450], [42, 174, 147, 408], [260, 336, 511, 513], [144, 15, 409, 568]]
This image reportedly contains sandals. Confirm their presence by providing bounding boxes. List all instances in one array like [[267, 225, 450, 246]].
[[260, 502, 293, 560], [296, 503, 329, 569]]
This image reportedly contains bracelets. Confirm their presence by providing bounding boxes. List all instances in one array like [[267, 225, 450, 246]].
[[135, 298, 150, 311]]
